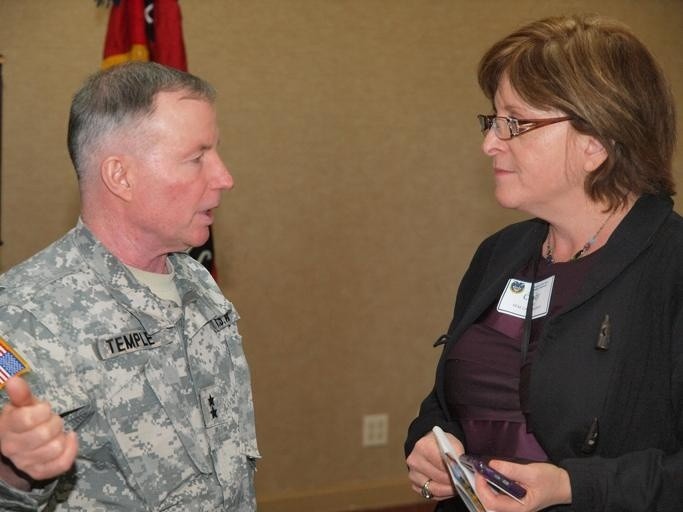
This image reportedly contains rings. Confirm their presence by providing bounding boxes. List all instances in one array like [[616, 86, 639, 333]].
[[422, 479, 433, 500]]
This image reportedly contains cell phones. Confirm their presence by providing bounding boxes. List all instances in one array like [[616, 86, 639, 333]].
[[459, 453, 528, 505]]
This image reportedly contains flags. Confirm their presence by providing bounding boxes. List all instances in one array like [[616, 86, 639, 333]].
[[101, 0, 218, 287]]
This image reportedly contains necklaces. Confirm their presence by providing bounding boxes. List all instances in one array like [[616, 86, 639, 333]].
[[545, 188, 631, 267]]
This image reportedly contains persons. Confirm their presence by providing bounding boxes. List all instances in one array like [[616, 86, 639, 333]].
[[0, 61, 272, 512], [403, 15, 683, 512]]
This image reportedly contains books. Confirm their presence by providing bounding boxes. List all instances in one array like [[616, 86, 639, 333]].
[[432, 425, 489, 512]]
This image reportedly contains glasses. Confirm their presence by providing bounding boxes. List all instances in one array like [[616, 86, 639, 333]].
[[477, 112, 580, 141]]
